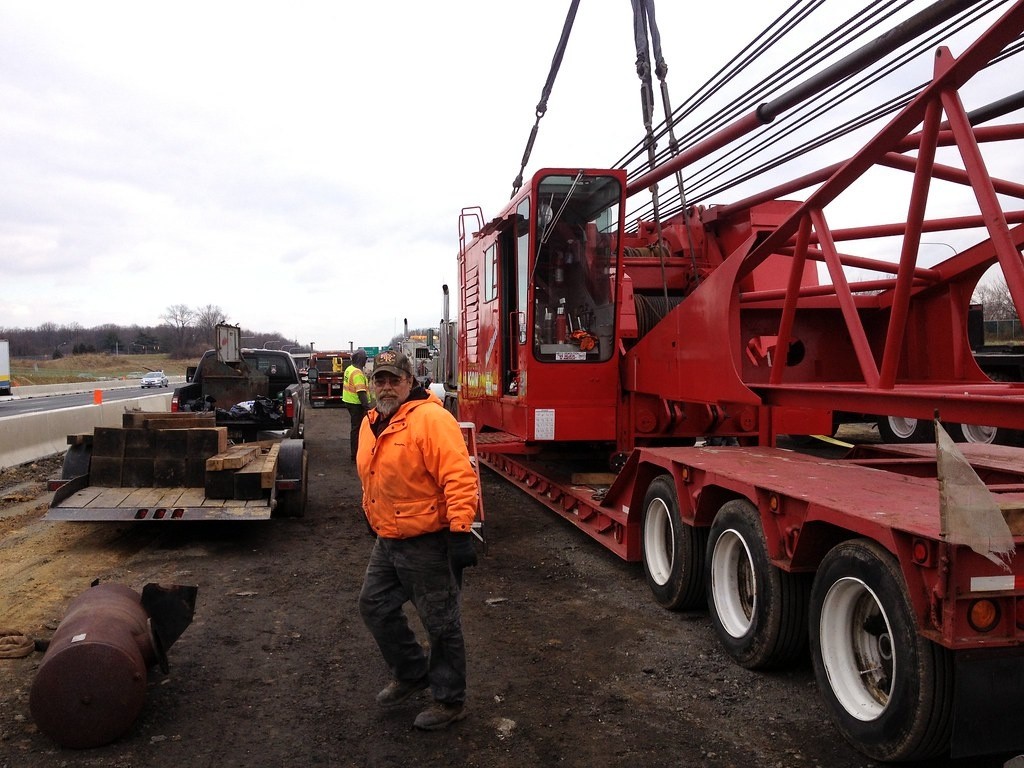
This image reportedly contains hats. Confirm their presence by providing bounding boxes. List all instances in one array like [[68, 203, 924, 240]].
[[371, 348, 413, 378]]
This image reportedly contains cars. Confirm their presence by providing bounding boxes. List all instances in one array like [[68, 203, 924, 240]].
[[140, 371, 168, 389], [76, 371, 142, 381]]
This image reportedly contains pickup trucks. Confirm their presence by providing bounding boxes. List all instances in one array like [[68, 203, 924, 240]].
[[171, 347, 304, 440]]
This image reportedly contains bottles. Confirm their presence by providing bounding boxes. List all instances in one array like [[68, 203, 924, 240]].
[[543, 313, 554, 344], [556, 307, 567, 344]]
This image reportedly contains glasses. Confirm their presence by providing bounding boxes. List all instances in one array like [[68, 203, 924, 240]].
[[374, 378, 408, 386]]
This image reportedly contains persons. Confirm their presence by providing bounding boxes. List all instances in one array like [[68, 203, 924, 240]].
[[355, 351, 488, 730], [342, 350, 373, 461]]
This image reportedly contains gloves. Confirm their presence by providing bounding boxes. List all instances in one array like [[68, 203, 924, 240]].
[[446, 537, 479, 568]]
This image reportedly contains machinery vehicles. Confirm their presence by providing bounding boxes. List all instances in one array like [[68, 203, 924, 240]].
[[309, 341, 354, 408], [385, 0, 1024, 768]]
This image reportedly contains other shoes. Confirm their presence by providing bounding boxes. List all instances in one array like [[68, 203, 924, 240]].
[[350, 457, 356, 463]]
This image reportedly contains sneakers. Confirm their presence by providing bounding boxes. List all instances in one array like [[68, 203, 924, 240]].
[[374, 671, 430, 706], [414, 702, 467, 730]]
